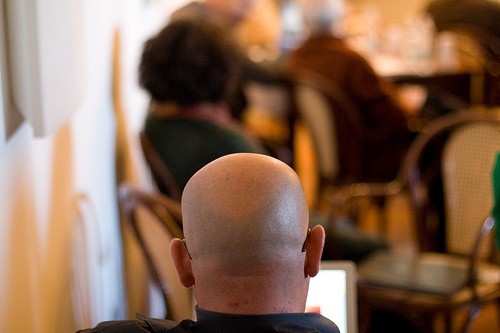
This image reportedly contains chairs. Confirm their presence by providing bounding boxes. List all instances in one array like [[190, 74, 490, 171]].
[[129, 198, 194, 318], [326, 106, 500, 333], [142, 86, 385, 242]]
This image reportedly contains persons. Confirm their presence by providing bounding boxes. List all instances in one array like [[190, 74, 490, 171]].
[[286, 6, 447, 211], [134, 18, 411, 332], [169, 0, 289, 122], [72, 149, 343, 332]]
